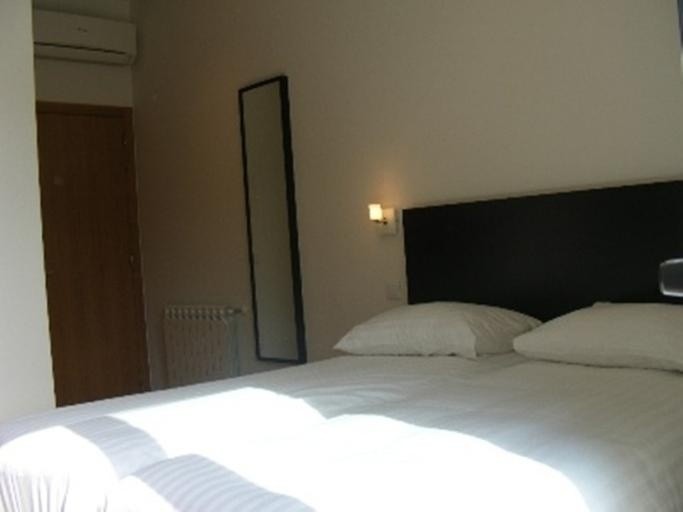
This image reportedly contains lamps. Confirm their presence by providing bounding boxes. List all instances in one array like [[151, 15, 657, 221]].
[[368, 203, 396, 240]]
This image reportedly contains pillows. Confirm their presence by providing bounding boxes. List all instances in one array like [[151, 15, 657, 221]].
[[332, 302, 544, 361], [512, 299, 683, 374]]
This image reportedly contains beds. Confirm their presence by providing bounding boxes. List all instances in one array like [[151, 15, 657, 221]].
[[0, 179, 683, 512]]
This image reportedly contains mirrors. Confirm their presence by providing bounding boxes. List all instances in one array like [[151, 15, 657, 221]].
[[238, 75, 307, 365]]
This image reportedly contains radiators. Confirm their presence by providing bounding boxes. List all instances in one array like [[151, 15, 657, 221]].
[[161, 303, 249, 388]]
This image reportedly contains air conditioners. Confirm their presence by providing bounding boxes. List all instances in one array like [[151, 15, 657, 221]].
[[33, 10, 138, 66]]
